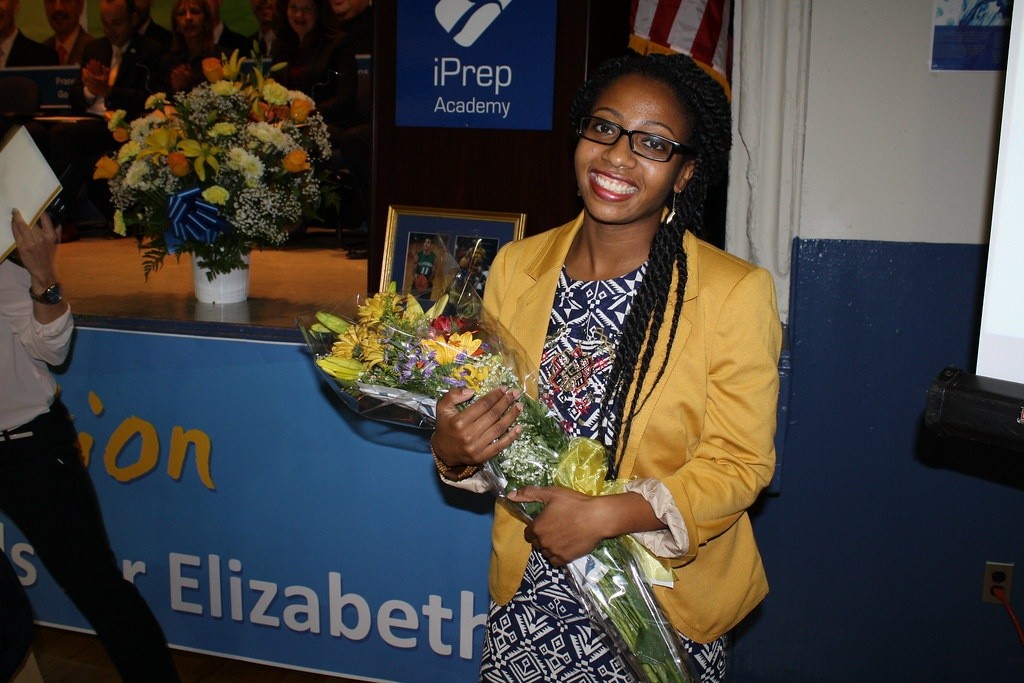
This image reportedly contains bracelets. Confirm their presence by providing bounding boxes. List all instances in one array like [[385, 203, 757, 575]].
[[430, 431, 478, 482]]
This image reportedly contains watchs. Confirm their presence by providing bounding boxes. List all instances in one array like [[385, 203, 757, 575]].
[[29, 282, 63, 305]]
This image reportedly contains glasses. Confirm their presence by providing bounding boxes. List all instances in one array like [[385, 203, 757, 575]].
[[576, 115, 697, 163]]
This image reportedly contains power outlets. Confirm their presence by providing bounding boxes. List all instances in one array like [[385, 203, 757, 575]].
[[982, 561, 1016, 605]]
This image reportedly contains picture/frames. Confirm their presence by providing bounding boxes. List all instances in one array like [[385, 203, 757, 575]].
[[378, 204, 526, 319]]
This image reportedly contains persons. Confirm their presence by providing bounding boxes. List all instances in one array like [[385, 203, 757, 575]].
[[429, 50, 783, 683], [165, 0, 248, 93], [458, 239, 487, 291], [249, 0, 372, 102], [0, 0, 172, 232], [0, 205, 173, 683], [412, 238, 437, 302]]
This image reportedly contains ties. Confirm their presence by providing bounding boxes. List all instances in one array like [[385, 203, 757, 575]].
[[108, 47, 122, 97], [57, 45, 67, 66], [258, 35, 267, 58]]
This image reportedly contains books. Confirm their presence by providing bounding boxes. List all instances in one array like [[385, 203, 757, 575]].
[[0, 124, 63, 264]]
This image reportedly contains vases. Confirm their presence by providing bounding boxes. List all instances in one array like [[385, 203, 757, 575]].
[[191, 250, 252, 304]]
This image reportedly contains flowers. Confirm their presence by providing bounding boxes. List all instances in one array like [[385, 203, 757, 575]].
[[92, 47, 332, 281], [292, 233, 702, 682]]
